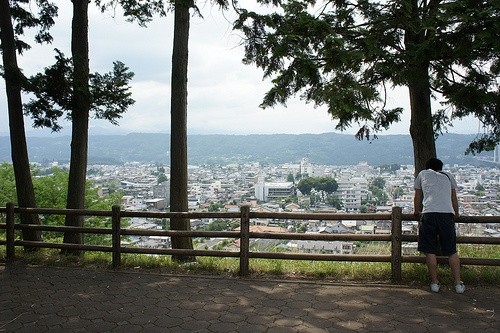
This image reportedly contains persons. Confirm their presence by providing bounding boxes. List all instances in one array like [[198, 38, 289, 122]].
[[414, 158, 466, 294]]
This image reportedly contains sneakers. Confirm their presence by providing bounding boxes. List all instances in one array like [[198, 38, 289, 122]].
[[455, 281, 466, 293], [430, 281, 441, 292]]
